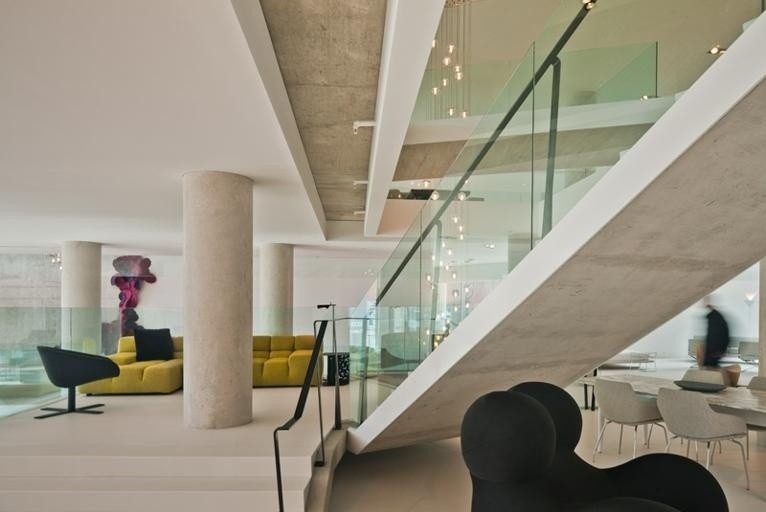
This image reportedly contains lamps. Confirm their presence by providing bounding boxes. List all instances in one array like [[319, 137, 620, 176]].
[[411, 0, 472, 357], [317, 303, 338, 354]]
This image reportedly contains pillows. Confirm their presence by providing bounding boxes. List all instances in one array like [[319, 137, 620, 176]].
[[133, 327, 174, 362]]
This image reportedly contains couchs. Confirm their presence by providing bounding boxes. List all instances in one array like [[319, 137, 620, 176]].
[[77, 334, 325, 396]]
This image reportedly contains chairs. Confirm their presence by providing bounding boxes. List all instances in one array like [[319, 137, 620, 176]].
[[460, 381, 728, 511], [737, 341, 760, 363], [32, 346, 120, 419], [589, 351, 765, 492], [687, 338, 706, 359]]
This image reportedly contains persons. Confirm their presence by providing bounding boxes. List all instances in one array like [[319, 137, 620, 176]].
[[696, 294, 731, 368]]
